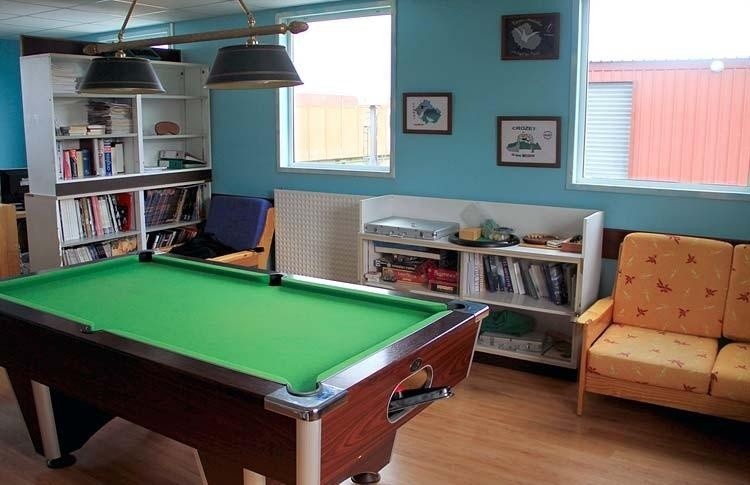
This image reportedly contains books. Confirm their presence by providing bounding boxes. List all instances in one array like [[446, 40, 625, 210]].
[[63, 139, 81, 150], [115, 141, 125, 173], [70, 150, 78, 178], [111, 147, 117, 176], [63, 151, 73, 180], [59, 234, 138, 268], [56, 139, 64, 181], [59, 99, 133, 136], [146, 223, 198, 251], [98, 137, 106, 177], [144, 182, 207, 228], [93, 138, 99, 177], [144, 165, 166, 174], [364, 239, 459, 294], [82, 150, 92, 177], [464, 253, 579, 309], [104, 138, 111, 177], [480, 326, 548, 353], [58, 192, 136, 239], [76, 151, 84, 177]]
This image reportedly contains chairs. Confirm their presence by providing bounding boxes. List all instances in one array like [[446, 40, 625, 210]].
[[159, 194, 275, 269]]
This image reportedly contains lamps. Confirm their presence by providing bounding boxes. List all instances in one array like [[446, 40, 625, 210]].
[[77, 0, 309, 95]]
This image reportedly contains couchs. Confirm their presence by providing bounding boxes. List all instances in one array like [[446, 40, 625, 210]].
[[575, 232, 749, 423]]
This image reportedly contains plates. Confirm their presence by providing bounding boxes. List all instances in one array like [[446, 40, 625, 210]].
[[522, 235, 557, 245], [447, 231, 520, 249]]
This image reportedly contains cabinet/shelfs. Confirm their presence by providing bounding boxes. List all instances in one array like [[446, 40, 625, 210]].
[[18, 52, 213, 196], [23, 179, 212, 273], [359, 194, 605, 369]]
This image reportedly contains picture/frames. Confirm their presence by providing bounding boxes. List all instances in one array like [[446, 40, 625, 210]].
[[500, 12, 560, 61], [402, 92, 453, 135], [497, 116, 562, 169]]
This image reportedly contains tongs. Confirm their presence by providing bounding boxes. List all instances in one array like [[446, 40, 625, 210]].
[[387, 386, 454, 415]]
